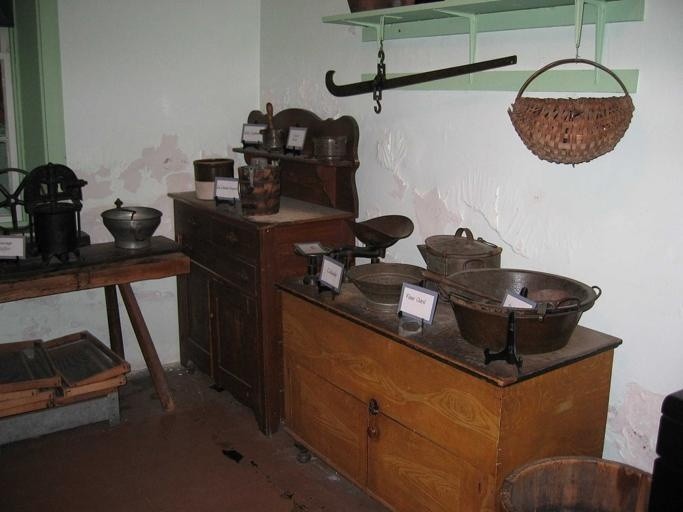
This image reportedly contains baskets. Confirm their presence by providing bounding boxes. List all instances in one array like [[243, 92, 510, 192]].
[[506, 58, 635, 166]]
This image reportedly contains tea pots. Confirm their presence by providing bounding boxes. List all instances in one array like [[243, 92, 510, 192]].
[[416, 226, 502, 293]]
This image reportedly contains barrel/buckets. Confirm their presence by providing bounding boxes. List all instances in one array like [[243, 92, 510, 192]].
[[33, 203, 76, 255]]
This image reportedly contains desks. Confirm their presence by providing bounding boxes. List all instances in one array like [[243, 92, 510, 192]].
[[0, 236, 191, 411]]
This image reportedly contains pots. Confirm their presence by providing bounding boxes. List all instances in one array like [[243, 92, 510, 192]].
[[343, 261, 429, 306], [101, 206, 163, 249], [445, 266, 603, 357]]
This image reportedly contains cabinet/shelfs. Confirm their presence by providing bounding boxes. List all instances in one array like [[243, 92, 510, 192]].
[[281, 290, 623, 511], [167, 192, 358, 439]]
